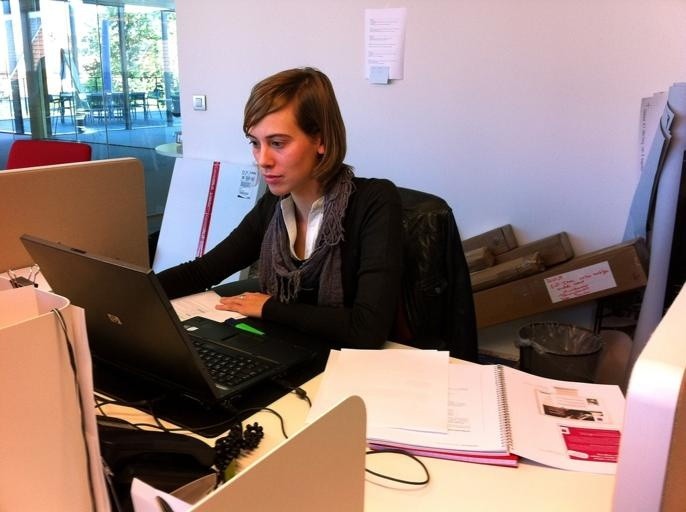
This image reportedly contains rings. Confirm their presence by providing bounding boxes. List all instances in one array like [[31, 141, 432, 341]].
[[240, 295, 244, 300]]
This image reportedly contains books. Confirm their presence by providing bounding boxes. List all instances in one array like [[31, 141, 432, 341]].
[[308, 349, 625, 475], [169, 289, 248, 324], [368, 443, 518, 468]]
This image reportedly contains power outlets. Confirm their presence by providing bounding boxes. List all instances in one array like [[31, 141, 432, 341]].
[[192, 95, 206, 112]]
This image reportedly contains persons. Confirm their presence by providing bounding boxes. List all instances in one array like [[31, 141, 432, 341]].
[[157, 68, 401, 349]]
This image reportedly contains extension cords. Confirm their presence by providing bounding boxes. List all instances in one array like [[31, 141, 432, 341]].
[[215, 429, 268, 472]]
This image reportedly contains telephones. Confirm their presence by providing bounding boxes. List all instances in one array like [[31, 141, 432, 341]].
[[95, 415, 265, 512]]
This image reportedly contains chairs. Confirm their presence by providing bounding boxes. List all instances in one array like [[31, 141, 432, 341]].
[[6, 140, 92, 170], [88, 94, 110, 122], [147, 89, 163, 122], [128, 92, 146, 128], [108, 92, 131, 127], [48, 94, 75, 133]]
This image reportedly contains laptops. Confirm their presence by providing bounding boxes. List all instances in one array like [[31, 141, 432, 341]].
[[20, 234, 331, 438]]
